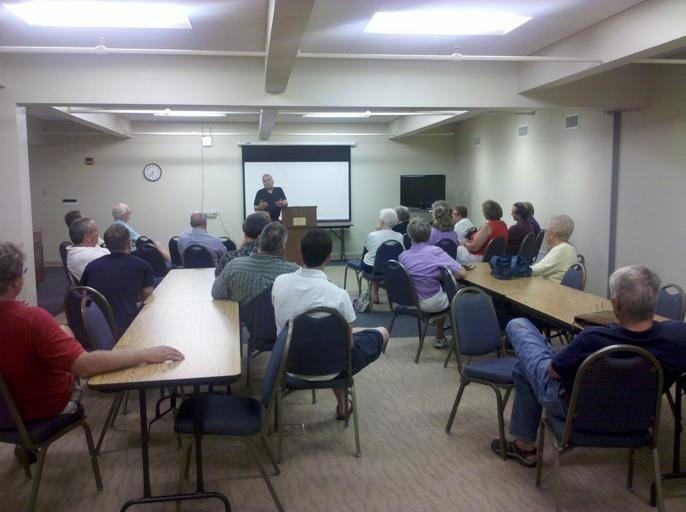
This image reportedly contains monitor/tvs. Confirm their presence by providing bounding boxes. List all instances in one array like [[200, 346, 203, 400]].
[[399, 174, 445, 212]]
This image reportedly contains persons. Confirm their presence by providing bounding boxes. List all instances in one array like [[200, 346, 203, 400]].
[[269, 226, 389, 419], [57, 204, 304, 357], [253, 173, 288, 222], [0, 237, 186, 465], [489, 264, 684, 468], [364, 198, 582, 348]]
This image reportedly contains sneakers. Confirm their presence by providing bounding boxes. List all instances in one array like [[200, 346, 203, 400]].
[[492, 439, 538, 466], [433, 335, 452, 348]]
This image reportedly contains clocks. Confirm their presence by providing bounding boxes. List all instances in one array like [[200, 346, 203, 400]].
[[142, 163, 161, 181]]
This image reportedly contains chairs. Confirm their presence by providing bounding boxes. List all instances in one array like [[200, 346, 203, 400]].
[[80, 297, 186, 454], [656, 281, 685, 324], [342, 222, 588, 364], [535, 345, 669, 512], [173, 319, 295, 512], [64, 286, 118, 353], [0, 374, 103, 510], [267, 306, 364, 461], [446, 284, 521, 459], [58, 236, 235, 287]]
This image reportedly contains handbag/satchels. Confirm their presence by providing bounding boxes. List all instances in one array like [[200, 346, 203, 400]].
[[464, 227, 477, 240], [489, 255, 532, 280]]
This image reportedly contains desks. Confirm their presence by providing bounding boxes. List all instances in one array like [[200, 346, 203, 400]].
[[89, 264, 241, 512], [454, 261, 685, 507]]
